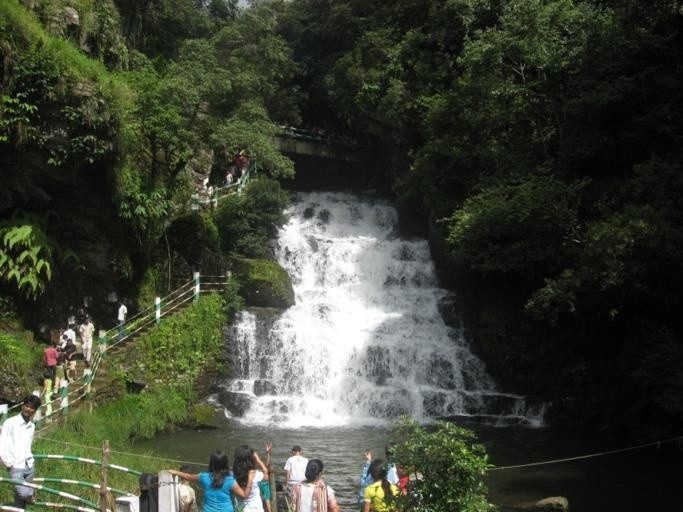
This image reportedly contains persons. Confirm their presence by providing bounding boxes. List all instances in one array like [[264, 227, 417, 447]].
[[205, 152, 248, 210], [40, 300, 128, 405], [167, 442, 411, 512], [0, 395, 42, 508]]
